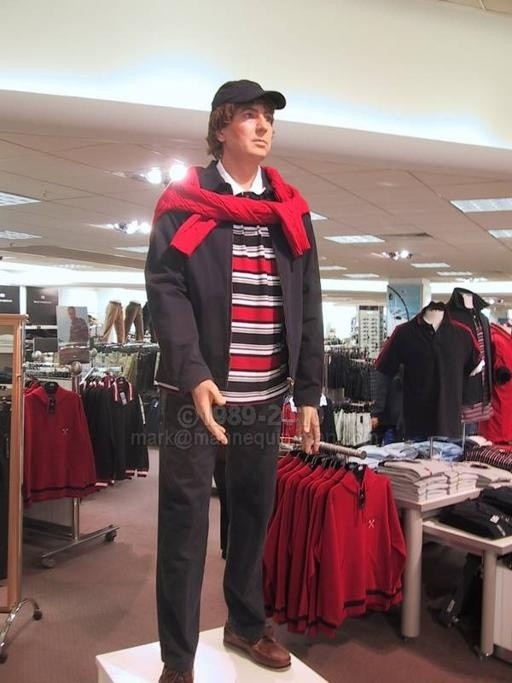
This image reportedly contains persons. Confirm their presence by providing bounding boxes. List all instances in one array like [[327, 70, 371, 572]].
[[142, 302, 158, 343], [68, 306, 89, 346], [445, 284, 494, 433], [487, 322, 512, 446], [100, 301, 125, 345], [124, 302, 144, 343], [372, 302, 483, 462], [145, 78, 324, 683]]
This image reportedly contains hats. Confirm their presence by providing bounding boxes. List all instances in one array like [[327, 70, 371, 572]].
[[212, 79, 286, 110]]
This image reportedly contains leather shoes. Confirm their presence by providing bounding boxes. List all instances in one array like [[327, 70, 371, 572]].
[[223, 622, 292, 670], [158, 664, 199, 683]]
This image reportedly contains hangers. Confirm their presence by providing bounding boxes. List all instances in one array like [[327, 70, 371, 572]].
[[289, 436, 367, 480], [24, 366, 127, 392]]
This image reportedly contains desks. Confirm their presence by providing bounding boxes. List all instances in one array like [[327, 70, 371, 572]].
[[391, 487, 483, 641], [422, 516, 512, 661]]
